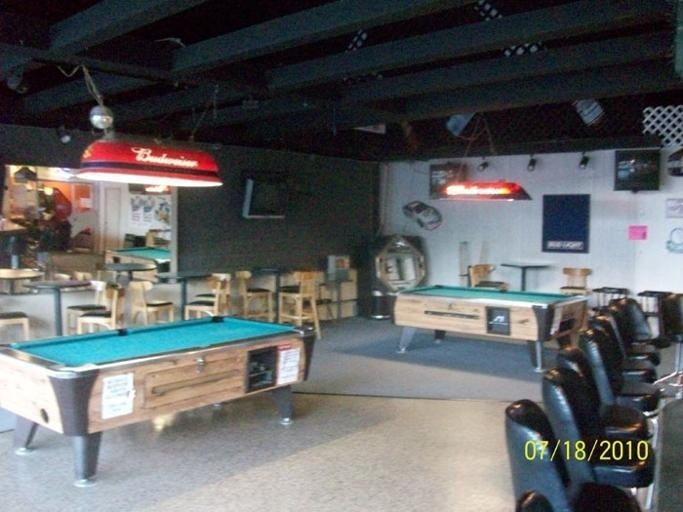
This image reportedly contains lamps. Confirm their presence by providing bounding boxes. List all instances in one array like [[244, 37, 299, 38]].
[[77, 82, 229, 193], [435, 110, 534, 203], [471, 149, 594, 174]]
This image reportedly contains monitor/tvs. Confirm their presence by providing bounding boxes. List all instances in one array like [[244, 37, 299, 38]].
[[615, 150, 660, 190]]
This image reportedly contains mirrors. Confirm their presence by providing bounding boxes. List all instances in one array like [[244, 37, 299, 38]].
[[1, 160, 179, 285]]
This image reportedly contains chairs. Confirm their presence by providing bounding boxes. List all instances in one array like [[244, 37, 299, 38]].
[[0, 262, 356, 344], [559, 267, 591, 295], [504, 286, 682, 512], [468, 265, 507, 294]]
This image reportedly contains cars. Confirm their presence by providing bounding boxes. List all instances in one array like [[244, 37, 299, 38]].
[[616, 155, 656, 183], [402, 200, 443, 231]]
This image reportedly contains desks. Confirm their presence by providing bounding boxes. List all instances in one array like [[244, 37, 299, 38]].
[[0, 312, 320, 488], [502, 263, 549, 293], [101, 244, 168, 282], [389, 283, 590, 374]]
